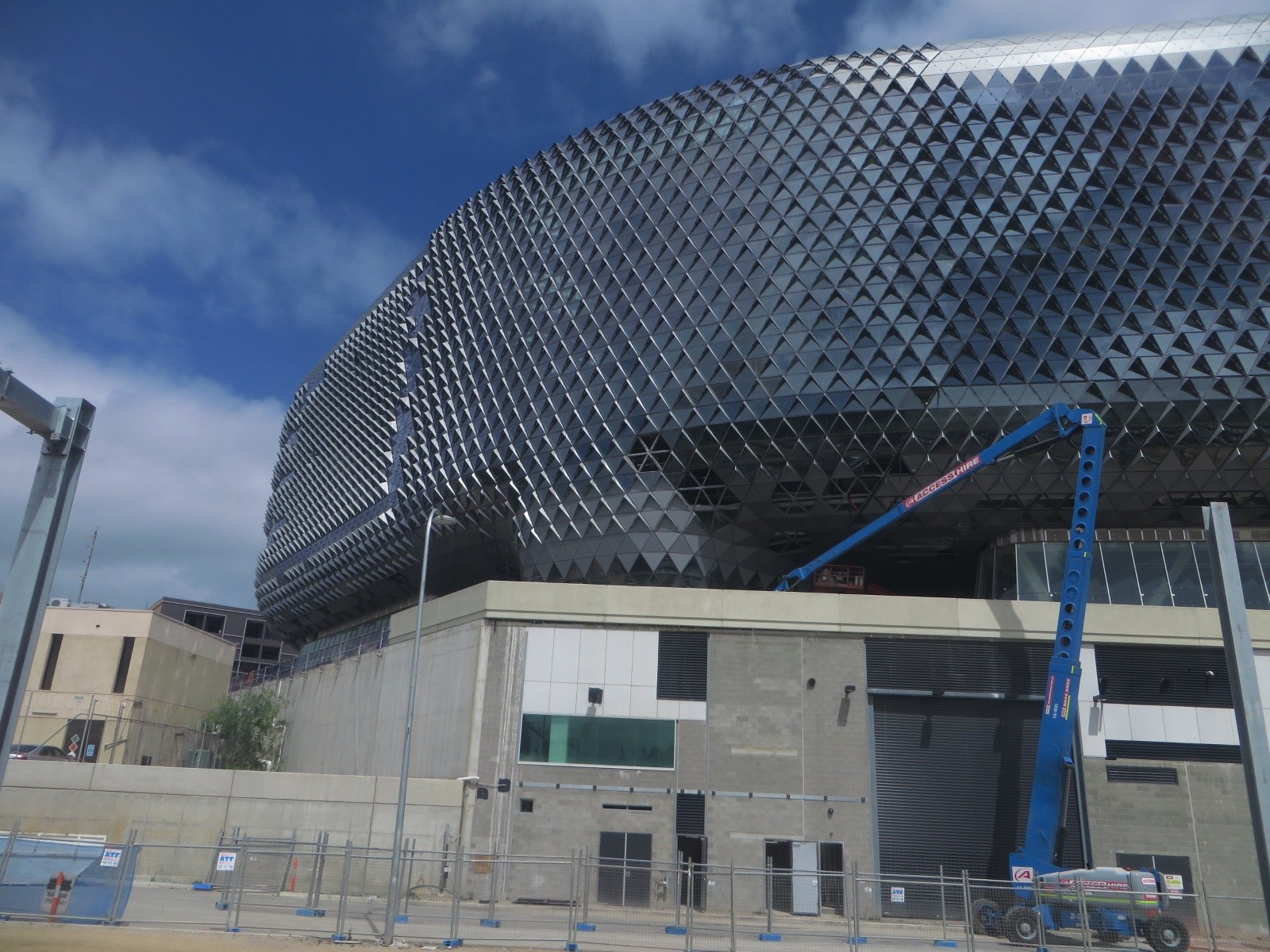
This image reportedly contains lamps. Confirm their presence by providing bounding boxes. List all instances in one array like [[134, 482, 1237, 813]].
[[844, 685, 856, 693], [1093, 694, 1109, 703], [587, 687, 603, 704], [1005, 692, 1019, 699], [932, 688, 945, 696]]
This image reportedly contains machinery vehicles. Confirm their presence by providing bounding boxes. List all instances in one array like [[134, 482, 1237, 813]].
[[775, 402, 1193, 952]]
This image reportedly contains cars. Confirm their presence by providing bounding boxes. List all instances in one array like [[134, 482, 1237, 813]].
[[8, 744, 76, 762]]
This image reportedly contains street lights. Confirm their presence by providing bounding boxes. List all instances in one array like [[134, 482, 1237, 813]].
[[381, 506, 458, 947]]
